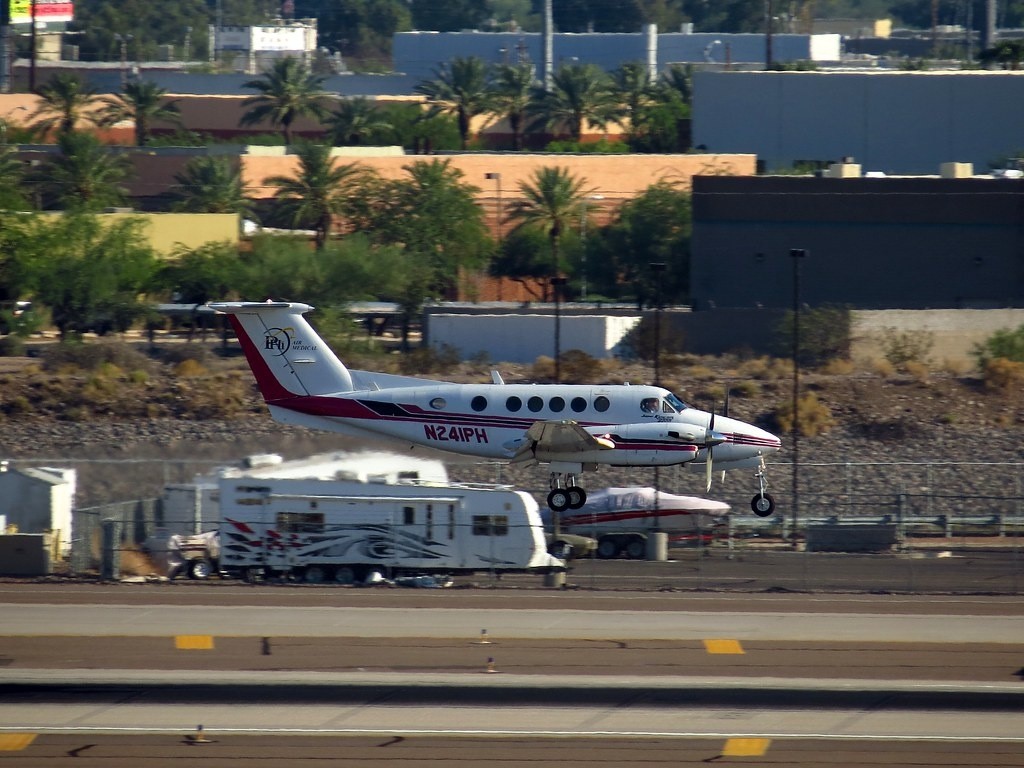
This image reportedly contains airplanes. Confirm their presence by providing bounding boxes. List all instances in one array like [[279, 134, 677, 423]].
[[149, 301, 782, 517], [541, 487, 732, 560]]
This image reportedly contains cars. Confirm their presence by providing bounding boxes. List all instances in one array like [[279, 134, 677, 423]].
[[545, 532, 599, 560]]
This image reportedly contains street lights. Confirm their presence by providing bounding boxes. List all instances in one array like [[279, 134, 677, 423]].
[[485, 172, 502, 300], [579, 194, 606, 300]]
